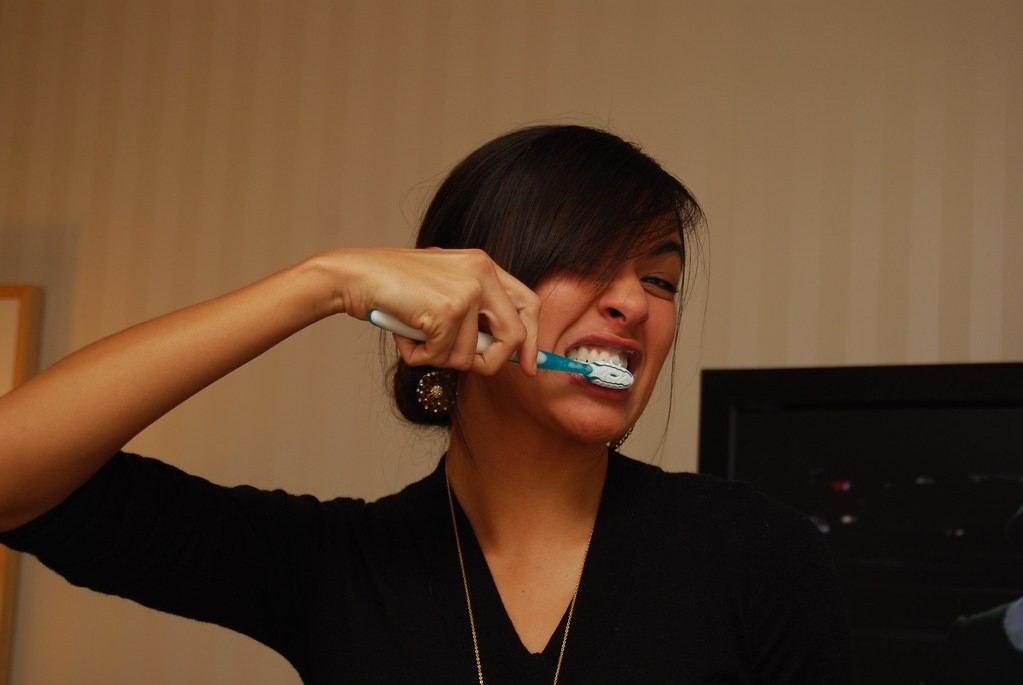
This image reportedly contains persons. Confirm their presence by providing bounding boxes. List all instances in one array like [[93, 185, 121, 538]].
[[0, 125, 855, 685]]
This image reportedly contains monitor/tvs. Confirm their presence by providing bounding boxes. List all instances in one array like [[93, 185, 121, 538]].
[[699, 361, 1022, 685]]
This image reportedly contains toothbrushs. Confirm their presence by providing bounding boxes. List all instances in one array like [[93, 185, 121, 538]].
[[369, 304, 640, 393]]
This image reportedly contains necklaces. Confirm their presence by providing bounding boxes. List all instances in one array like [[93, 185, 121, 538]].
[[445, 453, 595, 685]]
